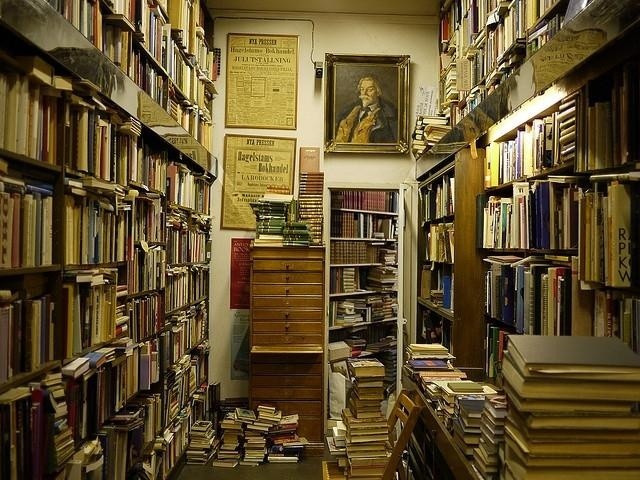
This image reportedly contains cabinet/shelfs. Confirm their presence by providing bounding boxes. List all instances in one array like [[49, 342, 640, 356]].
[[250, 244, 326, 457], [329, 209, 399, 406], [0, 0, 218, 480], [402, 0, 640, 480]]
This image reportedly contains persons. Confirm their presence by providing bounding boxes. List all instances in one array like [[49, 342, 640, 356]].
[[337, 71, 398, 145]]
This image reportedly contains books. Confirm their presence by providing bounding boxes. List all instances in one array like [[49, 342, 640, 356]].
[[1, 1, 217, 217], [1, 160, 218, 480], [401, 334, 640, 480], [248, 170, 325, 249], [330, 188, 398, 401], [217, 401, 312, 470], [325, 356, 398, 480], [402, 0, 640, 384]]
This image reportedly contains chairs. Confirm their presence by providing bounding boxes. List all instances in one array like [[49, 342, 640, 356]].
[[322, 388, 425, 480]]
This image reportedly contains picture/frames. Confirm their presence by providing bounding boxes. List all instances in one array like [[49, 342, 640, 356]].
[[324, 53, 410, 154]]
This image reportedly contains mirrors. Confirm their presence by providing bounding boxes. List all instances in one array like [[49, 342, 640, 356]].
[[323, 182, 404, 434]]
